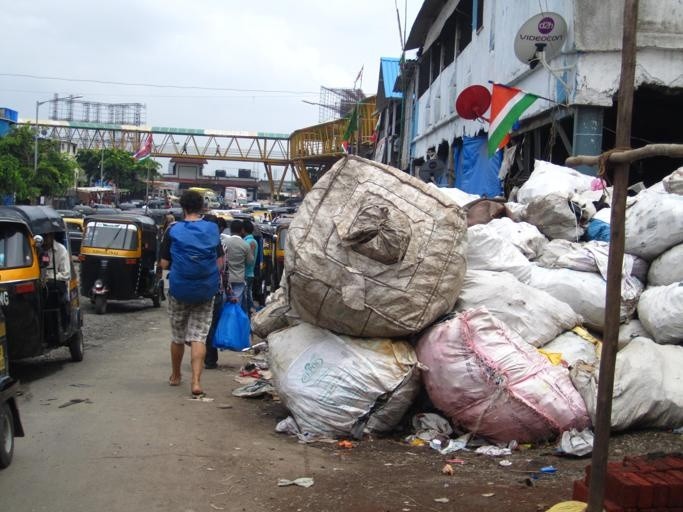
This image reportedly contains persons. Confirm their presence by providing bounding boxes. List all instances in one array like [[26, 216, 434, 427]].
[[39, 233, 70, 281], [180, 142, 187, 156], [214, 145, 221, 157], [159, 190, 222, 399], [163, 211, 262, 369]]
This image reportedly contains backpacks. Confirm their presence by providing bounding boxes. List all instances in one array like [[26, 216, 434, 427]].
[[169, 220, 220, 305]]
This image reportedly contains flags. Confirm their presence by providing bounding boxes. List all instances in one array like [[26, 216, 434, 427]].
[[129, 134, 151, 164], [369, 113, 380, 143], [488, 81, 538, 157], [339, 105, 357, 154]]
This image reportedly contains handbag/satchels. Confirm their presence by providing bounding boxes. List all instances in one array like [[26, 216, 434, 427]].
[[212, 296, 251, 352]]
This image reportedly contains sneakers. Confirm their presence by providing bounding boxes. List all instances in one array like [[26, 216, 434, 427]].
[[205, 363, 218, 369]]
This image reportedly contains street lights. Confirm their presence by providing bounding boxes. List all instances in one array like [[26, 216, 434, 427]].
[[34, 93, 83, 172], [145, 141, 180, 202], [98, 136, 135, 204]]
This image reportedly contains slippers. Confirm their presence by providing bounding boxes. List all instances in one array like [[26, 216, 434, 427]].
[[192, 392, 206, 399]]
